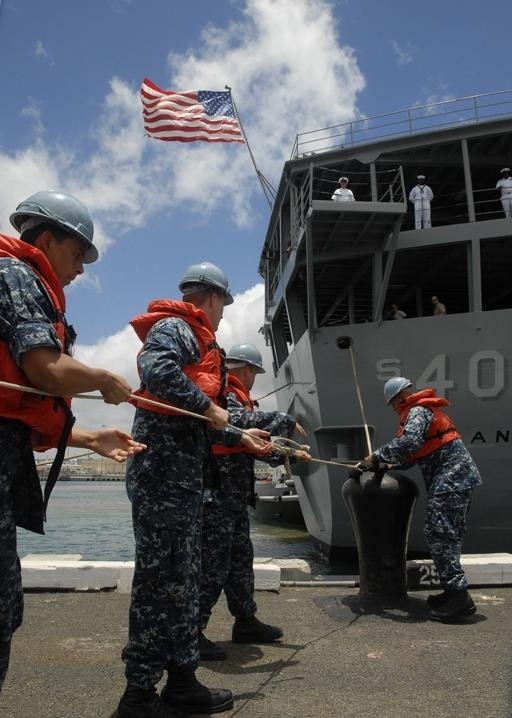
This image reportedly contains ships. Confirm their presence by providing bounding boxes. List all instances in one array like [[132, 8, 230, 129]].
[[251, 88, 512, 572]]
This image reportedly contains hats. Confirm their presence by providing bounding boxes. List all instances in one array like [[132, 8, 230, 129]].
[[416, 175, 427, 180], [338, 176, 350, 184]]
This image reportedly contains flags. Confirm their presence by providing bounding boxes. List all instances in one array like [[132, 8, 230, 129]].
[[140, 77, 246, 145]]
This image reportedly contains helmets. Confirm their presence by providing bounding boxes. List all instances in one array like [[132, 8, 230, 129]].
[[223, 341, 266, 376], [383, 375, 414, 407], [177, 260, 234, 307], [500, 167, 511, 174], [7, 189, 99, 266]]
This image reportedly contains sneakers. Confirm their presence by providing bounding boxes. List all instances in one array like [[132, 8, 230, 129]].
[[425, 585, 454, 605]]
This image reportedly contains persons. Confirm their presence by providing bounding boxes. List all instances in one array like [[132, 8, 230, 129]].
[[431, 295, 446, 315], [115, 258, 272, 717], [358, 376, 484, 621], [408, 174, 434, 229], [330, 176, 356, 201], [198, 341, 313, 662], [391, 303, 408, 319], [0, 187, 149, 694], [495, 167, 512, 219]]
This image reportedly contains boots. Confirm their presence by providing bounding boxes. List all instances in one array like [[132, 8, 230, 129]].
[[116, 682, 195, 718], [159, 666, 234, 715], [194, 629, 228, 662], [231, 614, 285, 645], [428, 589, 478, 622]]
